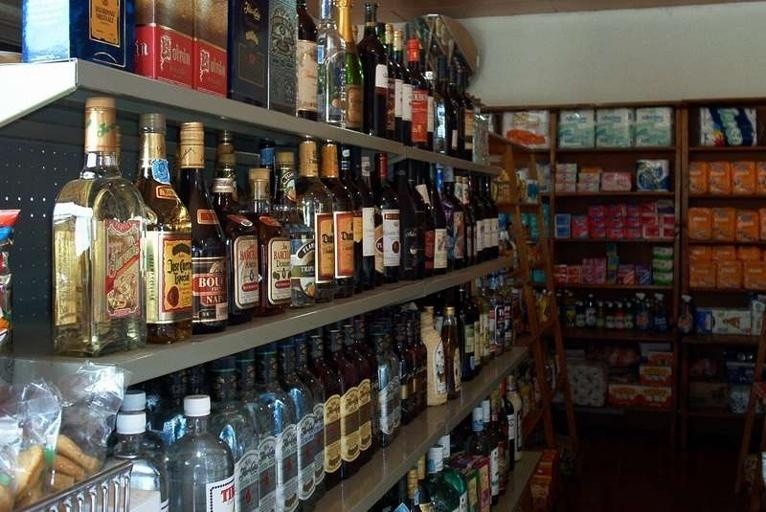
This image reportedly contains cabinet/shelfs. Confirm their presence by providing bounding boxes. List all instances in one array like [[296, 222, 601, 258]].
[[474, 93, 765, 425], [0, 55, 549, 511]]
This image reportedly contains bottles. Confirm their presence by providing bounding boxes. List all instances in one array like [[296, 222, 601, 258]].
[[555, 291, 694, 334], [520, 375, 541, 418], [294, 1, 491, 165], [48, 96, 525, 512]]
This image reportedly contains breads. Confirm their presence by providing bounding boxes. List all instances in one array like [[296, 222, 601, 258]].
[[0, 434, 97, 512]]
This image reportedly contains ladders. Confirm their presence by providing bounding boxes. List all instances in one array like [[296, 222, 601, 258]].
[[505, 146, 580, 473], [732, 312, 766, 509]]
